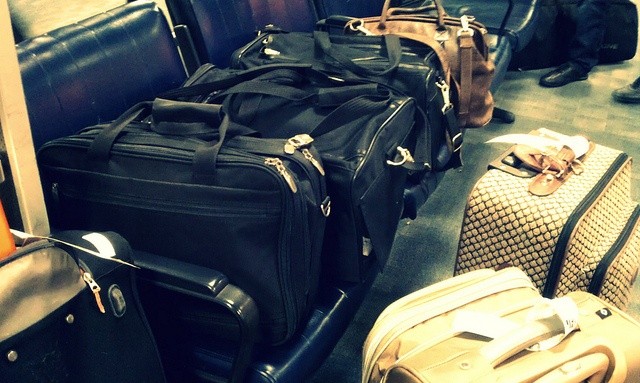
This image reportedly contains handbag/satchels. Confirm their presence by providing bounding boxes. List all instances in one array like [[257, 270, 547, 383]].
[[345, 0, 495, 127], [229, 22, 463, 170], [144, 60, 432, 281], [36, 97, 331, 345]]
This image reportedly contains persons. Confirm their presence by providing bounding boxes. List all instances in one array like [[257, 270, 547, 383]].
[[540, 0, 640, 103]]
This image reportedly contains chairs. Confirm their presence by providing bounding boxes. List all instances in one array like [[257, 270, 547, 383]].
[[313, 0, 512, 97], [0, 0, 378, 382], [438, 0, 542, 55], [165, 0, 448, 239]]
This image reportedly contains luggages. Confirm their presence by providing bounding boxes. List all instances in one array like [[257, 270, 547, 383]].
[[362, 265, 640, 383], [0, 228, 166, 382], [453, 127, 640, 311]]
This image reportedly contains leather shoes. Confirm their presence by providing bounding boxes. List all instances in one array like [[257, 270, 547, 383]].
[[612, 75, 640, 103], [540, 61, 588, 86]]
